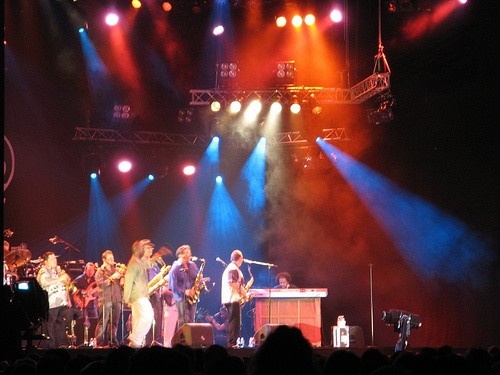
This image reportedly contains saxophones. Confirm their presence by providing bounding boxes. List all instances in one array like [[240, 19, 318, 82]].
[[186, 256, 206, 305], [148, 257, 172, 296], [237, 264, 254, 306]]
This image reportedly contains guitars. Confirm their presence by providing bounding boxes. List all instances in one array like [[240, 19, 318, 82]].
[[73, 281, 98, 309]]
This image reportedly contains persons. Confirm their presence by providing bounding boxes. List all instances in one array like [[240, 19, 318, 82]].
[[274, 272, 297, 289], [168, 246, 199, 323], [3, 239, 174, 348], [221, 250, 244, 348], [0, 324, 500, 375], [206, 307, 229, 345]]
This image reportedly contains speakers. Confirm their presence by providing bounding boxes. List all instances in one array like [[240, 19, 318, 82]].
[[350, 325, 365, 347], [171, 323, 214, 349], [253, 323, 288, 348]]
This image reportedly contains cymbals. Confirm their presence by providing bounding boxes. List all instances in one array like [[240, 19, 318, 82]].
[[4, 249, 31, 268]]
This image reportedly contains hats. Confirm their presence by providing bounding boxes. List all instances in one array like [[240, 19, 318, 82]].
[[176, 244, 189, 256], [139, 239, 155, 246]]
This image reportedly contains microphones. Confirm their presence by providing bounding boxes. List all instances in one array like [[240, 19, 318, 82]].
[[215, 256, 227, 267], [94, 262, 99, 271], [286, 284, 290, 289], [48, 237, 60, 244]]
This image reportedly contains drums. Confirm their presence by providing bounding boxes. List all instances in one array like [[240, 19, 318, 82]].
[[22, 259, 45, 278]]
